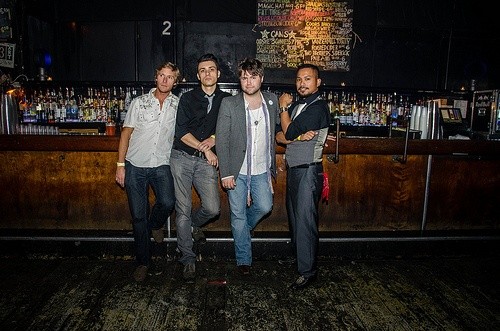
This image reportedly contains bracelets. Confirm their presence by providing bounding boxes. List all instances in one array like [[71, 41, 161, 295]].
[[297, 134, 301, 141], [116, 162, 125, 167], [210, 135, 215, 139]]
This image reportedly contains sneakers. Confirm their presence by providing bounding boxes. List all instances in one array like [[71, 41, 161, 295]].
[[134, 265, 149, 281], [183, 260, 196, 280], [152, 226, 164, 243], [191, 225, 207, 246]]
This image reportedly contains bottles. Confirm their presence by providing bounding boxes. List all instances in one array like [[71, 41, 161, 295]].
[[265, 88, 301, 127], [321, 91, 467, 139], [0, 68, 149, 134]]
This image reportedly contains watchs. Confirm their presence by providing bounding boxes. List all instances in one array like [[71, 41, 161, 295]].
[[279, 107, 288, 114]]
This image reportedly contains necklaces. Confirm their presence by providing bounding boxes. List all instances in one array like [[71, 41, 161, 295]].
[[248, 103, 261, 125]]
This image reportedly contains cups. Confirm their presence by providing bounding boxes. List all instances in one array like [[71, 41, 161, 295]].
[[106, 123, 115, 136]]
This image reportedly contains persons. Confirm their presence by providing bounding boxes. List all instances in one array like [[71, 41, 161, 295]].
[[170, 53, 233, 283], [116, 63, 179, 281], [274, 63, 330, 290], [215, 55, 336, 274]]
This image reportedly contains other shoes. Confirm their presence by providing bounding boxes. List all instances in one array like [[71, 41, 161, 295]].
[[278, 255, 298, 266], [294, 271, 318, 288], [239, 265, 251, 275]]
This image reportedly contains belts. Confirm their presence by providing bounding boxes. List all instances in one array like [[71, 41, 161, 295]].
[[288, 161, 322, 169]]
